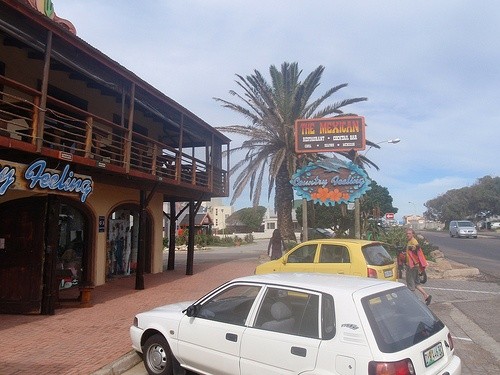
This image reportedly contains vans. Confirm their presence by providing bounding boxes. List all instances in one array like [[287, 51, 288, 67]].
[[448, 220, 478, 239]]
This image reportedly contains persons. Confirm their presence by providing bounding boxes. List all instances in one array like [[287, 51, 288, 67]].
[[404, 227, 432, 306], [268, 229, 285, 260]]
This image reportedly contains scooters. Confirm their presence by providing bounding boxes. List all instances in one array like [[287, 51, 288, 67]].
[[393, 243, 427, 285]]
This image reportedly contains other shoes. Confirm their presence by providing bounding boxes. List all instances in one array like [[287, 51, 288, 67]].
[[425, 295, 432, 305]]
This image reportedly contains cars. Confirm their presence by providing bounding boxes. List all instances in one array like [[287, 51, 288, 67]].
[[299, 227, 346, 242], [252, 237, 399, 305], [129, 272, 463, 375]]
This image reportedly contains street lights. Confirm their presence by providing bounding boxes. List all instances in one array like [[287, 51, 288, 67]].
[[363, 138, 401, 157]]
